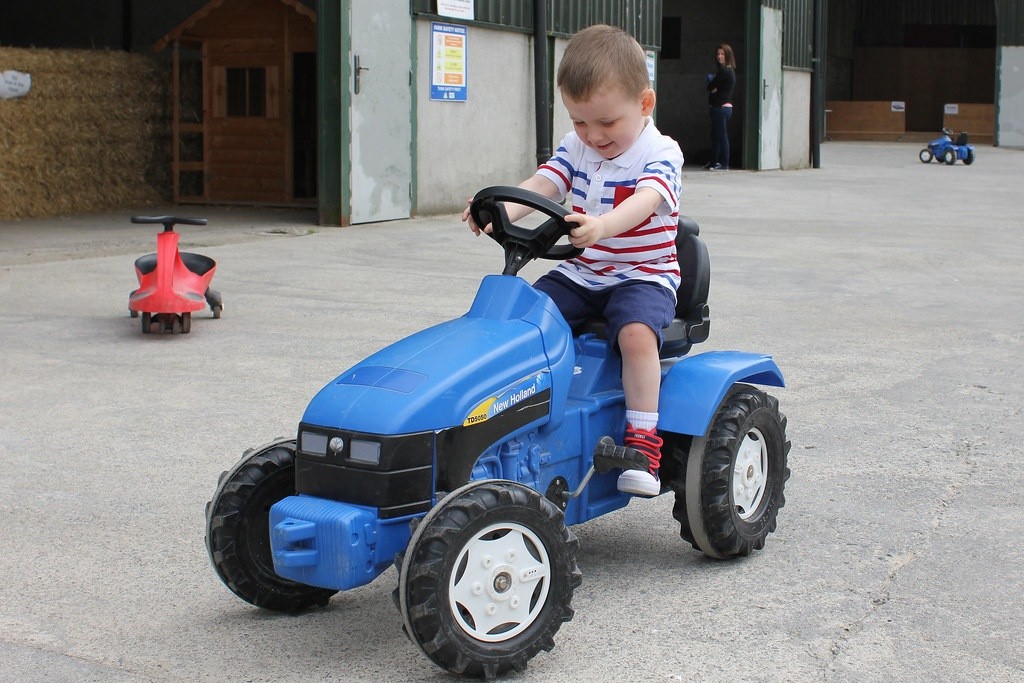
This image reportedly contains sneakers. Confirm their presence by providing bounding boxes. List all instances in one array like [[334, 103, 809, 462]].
[[709, 163, 730, 171], [617, 422, 664, 496], [702, 162, 719, 169]]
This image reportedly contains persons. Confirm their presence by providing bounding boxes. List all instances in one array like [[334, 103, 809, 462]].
[[703, 43, 735, 171], [463, 25, 681, 496]]
